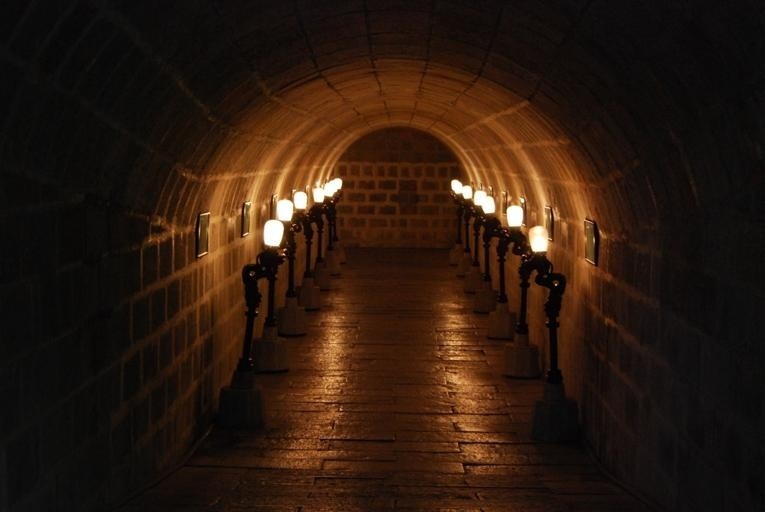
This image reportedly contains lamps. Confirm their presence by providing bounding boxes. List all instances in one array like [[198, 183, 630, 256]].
[[445, 177, 582, 443], [217, 177, 344, 431]]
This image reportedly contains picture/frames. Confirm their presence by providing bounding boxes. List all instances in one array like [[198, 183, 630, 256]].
[[584, 220, 599, 266], [196, 212, 211, 258]]
